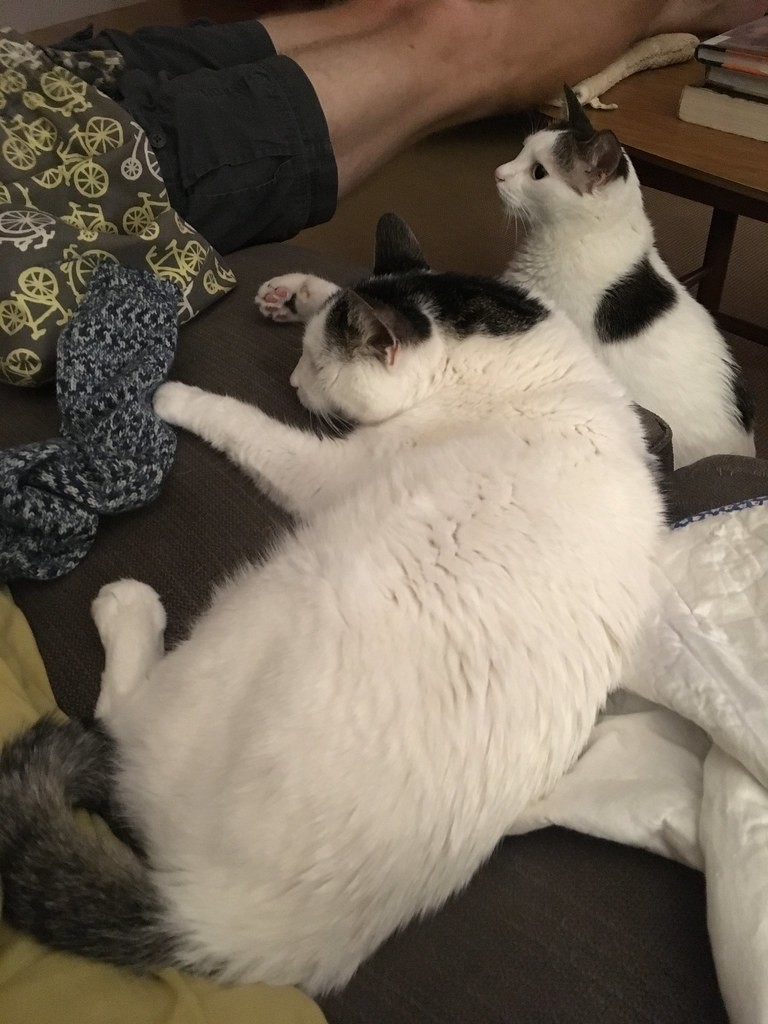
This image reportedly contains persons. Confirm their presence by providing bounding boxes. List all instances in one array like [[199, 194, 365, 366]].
[[0, 0, 768, 390]]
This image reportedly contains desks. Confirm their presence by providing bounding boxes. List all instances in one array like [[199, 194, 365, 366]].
[[537, 38, 768, 346]]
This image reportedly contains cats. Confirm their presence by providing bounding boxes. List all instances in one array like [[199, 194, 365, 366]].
[[1, 211, 670, 1004], [480, 79, 757, 471]]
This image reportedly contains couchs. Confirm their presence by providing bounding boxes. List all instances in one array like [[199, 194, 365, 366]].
[[0, 243, 730, 1024]]
